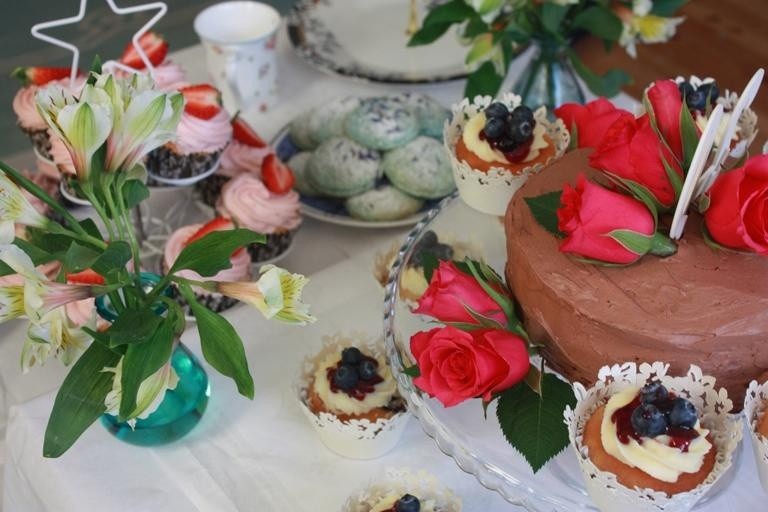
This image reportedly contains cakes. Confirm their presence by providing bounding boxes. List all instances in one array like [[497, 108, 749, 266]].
[[296, 332, 416, 460], [674, 73, 759, 158], [505, 142, 768, 412], [744, 380, 768, 494], [370, 229, 488, 362], [563, 359, 744, 512], [341, 467, 462, 512], [441, 92, 571, 216]]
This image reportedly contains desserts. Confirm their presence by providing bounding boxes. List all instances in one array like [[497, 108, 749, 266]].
[[0, 31, 304, 333]]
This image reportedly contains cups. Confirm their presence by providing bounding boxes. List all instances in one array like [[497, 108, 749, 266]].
[[194, 0, 282, 114]]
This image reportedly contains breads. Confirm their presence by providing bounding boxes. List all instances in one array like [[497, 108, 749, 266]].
[[289, 90, 457, 221]]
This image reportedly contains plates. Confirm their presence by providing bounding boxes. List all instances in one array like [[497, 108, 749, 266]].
[[266, 86, 460, 229], [286, 1, 534, 85]]
